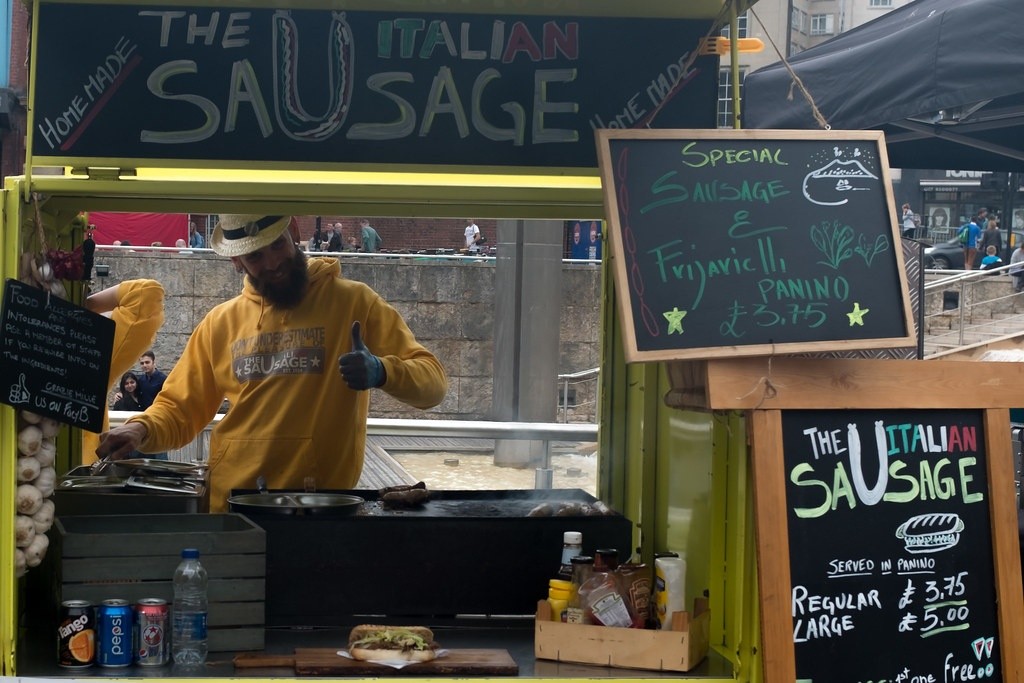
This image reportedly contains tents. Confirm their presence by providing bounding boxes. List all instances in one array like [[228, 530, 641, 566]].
[[744, 1, 1024, 170]]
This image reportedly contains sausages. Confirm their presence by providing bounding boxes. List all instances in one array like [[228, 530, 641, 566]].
[[526, 500, 615, 517], [380, 481, 428, 504]]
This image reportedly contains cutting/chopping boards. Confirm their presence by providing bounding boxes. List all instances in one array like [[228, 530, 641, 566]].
[[234, 648, 518, 676]]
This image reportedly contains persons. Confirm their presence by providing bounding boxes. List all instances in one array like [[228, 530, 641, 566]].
[[112, 222, 204, 248], [96, 211, 447, 513], [82, 278, 165, 463], [463, 219, 485, 253], [957, 208, 1024, 291], [357, 218, 382, 254], [308, 222, 357, 253], [901, 203, 919, 238], [113, 352, 169, 461]]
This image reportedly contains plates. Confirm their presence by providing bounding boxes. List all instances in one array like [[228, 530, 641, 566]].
[[228, 490, 363, 516]]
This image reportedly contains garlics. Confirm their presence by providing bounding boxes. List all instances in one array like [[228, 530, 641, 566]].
[[12, 408, 60, 571]]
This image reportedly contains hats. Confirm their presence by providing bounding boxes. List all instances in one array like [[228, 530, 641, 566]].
[[210, 213, 291, 257]]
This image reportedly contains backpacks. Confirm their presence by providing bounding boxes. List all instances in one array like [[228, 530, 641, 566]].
[[903, 213, 921, 228], [194, 231, 204, 246]]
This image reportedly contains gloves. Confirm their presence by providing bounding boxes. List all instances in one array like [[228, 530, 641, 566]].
[[338, 321, 384, 391]]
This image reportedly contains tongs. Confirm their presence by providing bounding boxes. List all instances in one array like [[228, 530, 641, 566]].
[[89, 453, 110, 475], [111, 461, 208, 479]]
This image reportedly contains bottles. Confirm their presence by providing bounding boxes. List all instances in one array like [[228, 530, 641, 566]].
[[652, 551, 687, 631], [547, 579, 580, 622], [571, 549, 618, 625], [557, 531, 582, 580], [618, 564, 652, 629], [170, 548, 208, 665]]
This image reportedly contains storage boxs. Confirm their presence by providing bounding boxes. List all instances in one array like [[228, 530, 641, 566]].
[[51, 513, 265, 651], [535, 596, 711, 671]]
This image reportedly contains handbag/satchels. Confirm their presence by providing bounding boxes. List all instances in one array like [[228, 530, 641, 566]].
[[958, 227, 969, 244], [472, 225, 487, 245]]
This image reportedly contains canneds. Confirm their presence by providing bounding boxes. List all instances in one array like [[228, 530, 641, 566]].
[[95, 599, 133, 668], [133, 598, 172, 667], [55, 600, 95, 669]]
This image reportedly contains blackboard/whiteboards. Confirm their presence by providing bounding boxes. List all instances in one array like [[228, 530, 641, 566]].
[[595, 126, 918, 365], [705, 359, 1024, 683], [26, 1, 723, 176], [0, 278, 116, 433]]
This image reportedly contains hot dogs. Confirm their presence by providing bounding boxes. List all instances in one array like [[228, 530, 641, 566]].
[[348, 623, 439, 662]]
